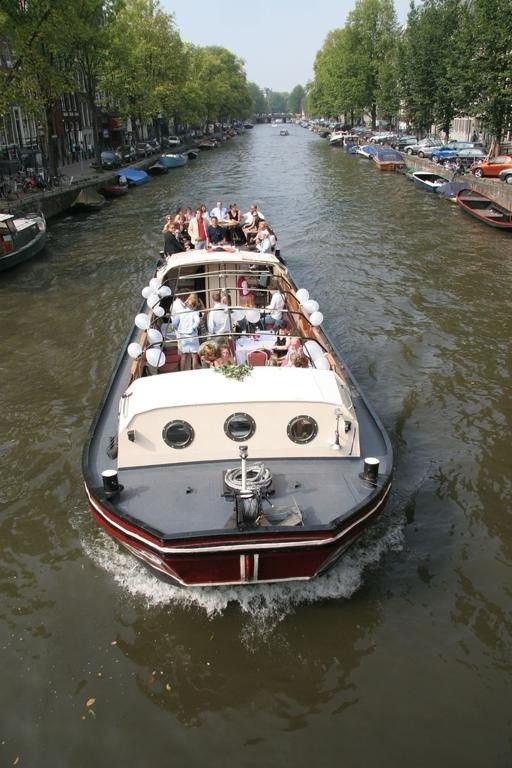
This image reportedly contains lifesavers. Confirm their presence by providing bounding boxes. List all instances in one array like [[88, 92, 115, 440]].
[[207, 247, 236, 254]]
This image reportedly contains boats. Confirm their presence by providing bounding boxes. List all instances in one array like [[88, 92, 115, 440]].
[[115, 168, 150, 187], [66, 187, 107, 213], [198, 121, 255, 148], [187, 148, 201, 159], [102, 180, 130, 197], [76, 201, 399, 590], [456, 184, 512, 230], [147, 161, 170, 176], [159, 152, 190, 169], [270, 115, 405, 173], [407, 168, 450, 192], [0, 209, 52, 272]]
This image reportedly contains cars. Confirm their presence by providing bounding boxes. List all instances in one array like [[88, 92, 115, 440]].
[[389, 132, 512, 183], [89, 135, 182, 170], [190, 129, 204, 138]]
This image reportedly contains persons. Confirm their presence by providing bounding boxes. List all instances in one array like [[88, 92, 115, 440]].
[[477, 131, 483, 142], [454, 156, 465, 176], [66, 140, 95, 164], [160, 278, 311, 372], [471, 131, 478, 142], [162, 200, 279, 256]]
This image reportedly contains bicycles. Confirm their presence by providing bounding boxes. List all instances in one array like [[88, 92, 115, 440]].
[[0, 160, 73, 200]]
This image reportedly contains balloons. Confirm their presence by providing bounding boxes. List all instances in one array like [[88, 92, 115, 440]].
[[149, 277, 162, 290], [134, 312, 151, 331], [142, 286, 156, 299], [310, 311, 324, 327], [302, 299, 319, 314], [157, 285, 172, 297], [127, 342, 144, 360], [147, 295, 160, 308], [146, 348, 166, 369], [148, 328, 165, 349], [153, 306, 166, 318]]
